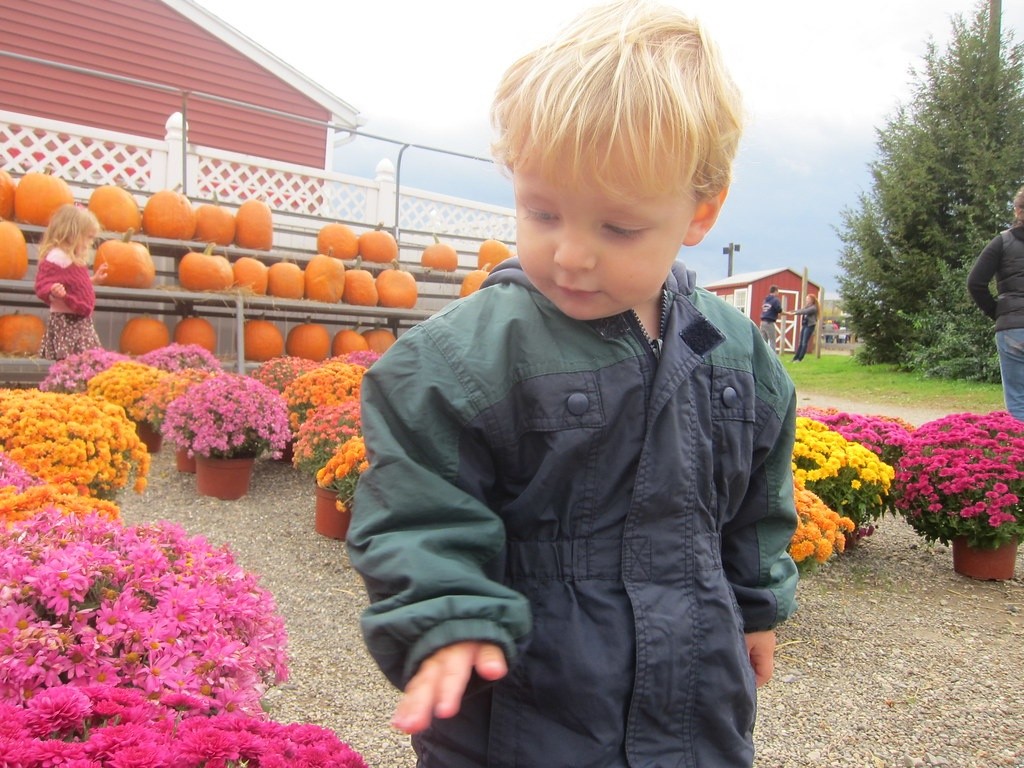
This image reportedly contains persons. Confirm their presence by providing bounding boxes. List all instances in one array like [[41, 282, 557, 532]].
[[789, 295, 821, 364], [822, 319, 858, 343], [760, 285, 790, 352], [968, 184, 1024, 422], [345, 0, 797, 768], [35, 202, 108, 364]]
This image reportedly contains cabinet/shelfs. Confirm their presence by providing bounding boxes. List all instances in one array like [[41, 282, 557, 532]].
[[0, 199, 517, 375]]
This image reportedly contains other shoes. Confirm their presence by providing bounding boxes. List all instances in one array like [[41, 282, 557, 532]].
[[786, 359, 800, 363]]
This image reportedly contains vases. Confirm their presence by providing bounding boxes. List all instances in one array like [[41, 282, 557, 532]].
[[277, 439, 297, 462], [174, 441, 196, 473], [951, 538, 1018, 579], [194, 454, 255, 500], [129, 418, 163, 453], [315, 486, 352, 542]]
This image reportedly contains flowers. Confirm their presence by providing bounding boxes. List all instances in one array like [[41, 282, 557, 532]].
[[0, 343, 1024, 768]]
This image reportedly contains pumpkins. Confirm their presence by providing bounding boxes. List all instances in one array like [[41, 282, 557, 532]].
[[0, 167, 515, 365]]
